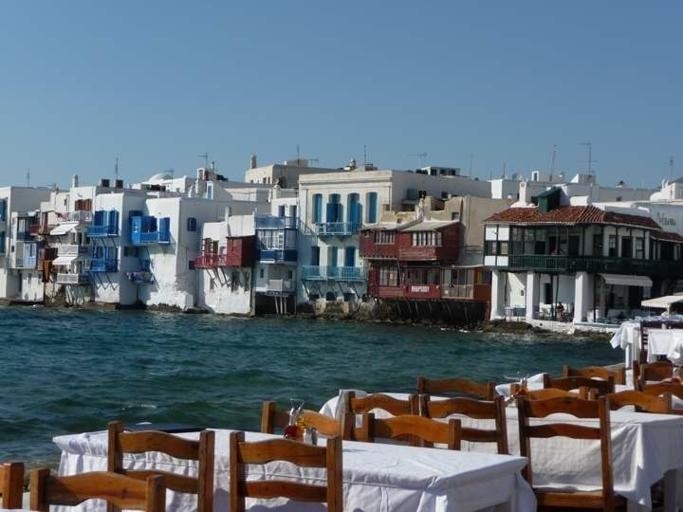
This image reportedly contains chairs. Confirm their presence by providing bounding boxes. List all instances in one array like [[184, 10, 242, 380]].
[[1, 458, 27, 510], [409, 392, 510, 456], [514, 392, 628, 510], [257, 399, 359, 442], [24, 465, 169, 511], [408, 320, 682, 421], [226, 429, 346, 511], [102, 421, 218, 511], [335, 389, 418, 446], [355, 409, 460, 452]]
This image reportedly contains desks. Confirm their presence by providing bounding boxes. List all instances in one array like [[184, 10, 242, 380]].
[[321, 384, 680, 511], [48, 425, 541, 512]]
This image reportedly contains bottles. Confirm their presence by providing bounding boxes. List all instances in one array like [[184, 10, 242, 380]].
[[518, 377, 528, 396], [289, 399, 306, 443]]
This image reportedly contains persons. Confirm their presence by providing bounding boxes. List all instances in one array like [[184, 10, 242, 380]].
[[616, 311, 625, 319], [555, 301, 565, 321]]
